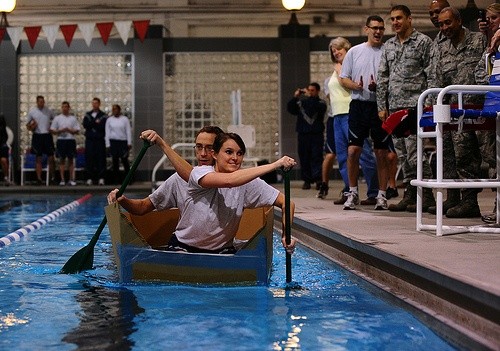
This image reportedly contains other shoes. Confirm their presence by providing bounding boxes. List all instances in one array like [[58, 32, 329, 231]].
[[334, 191, 348, 204], [302, 183, 310, 189], [361, 197, 377, 205], [71, 181, 76, 186], [316, 184, 321, 190], [87, 179, 92, 185], [99, 179, 104, 185], [60, 182, 65, 186]]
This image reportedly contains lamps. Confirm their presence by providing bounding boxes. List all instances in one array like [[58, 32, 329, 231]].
[[0, 0, 17, 27], [278, 0, 310, 38]]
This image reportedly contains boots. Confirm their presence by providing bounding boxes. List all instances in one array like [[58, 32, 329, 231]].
[[388, 186, 417, 211], [428, 188, 461, 215], [446, 189, 482, 218], [407, 187, 436, 212]]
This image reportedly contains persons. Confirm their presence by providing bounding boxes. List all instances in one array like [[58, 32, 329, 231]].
[[429, 0, 450, 28], [287, 83, 327, 190], [328, 37, 379, 205], [82, 98, 108, 185], [50, 101, 80, 185], [376, 5, 433, 212], [107, 126, 225, 219], [338, 15, 388, 211], [0, 112, 9, 181], [105, 104, 132, 186], [315, 76, 336, 200], [429, 7, 486, 220], [27, 95, 56, 186], [385, 141, 399, 199], [475, 2, 500, 223], [168, 132, 296, 256]]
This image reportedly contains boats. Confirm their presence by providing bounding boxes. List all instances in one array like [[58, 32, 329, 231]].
[[104, 201, 276, 284]]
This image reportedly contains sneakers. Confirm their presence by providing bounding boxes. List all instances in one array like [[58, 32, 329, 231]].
[[386, 186, 398, 199], [316, 183, 329, 198], [373, 194, 388, 210], [343, 191, 359, 210]]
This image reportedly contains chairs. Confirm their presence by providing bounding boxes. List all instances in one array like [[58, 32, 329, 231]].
[[72, 143, 86, 182], [228, 125, 264, 170], [21, 144, 51, 185]]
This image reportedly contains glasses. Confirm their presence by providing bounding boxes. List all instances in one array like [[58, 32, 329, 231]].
[[193, 145, 214, 154], [366, 25, 384, 31]]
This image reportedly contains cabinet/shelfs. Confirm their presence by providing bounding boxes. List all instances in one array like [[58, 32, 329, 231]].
[[410, 86, 500, 236]]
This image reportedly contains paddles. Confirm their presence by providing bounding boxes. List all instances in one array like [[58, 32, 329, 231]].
[[281, 169, 297, 287], [60, 139, 151, 271]]
